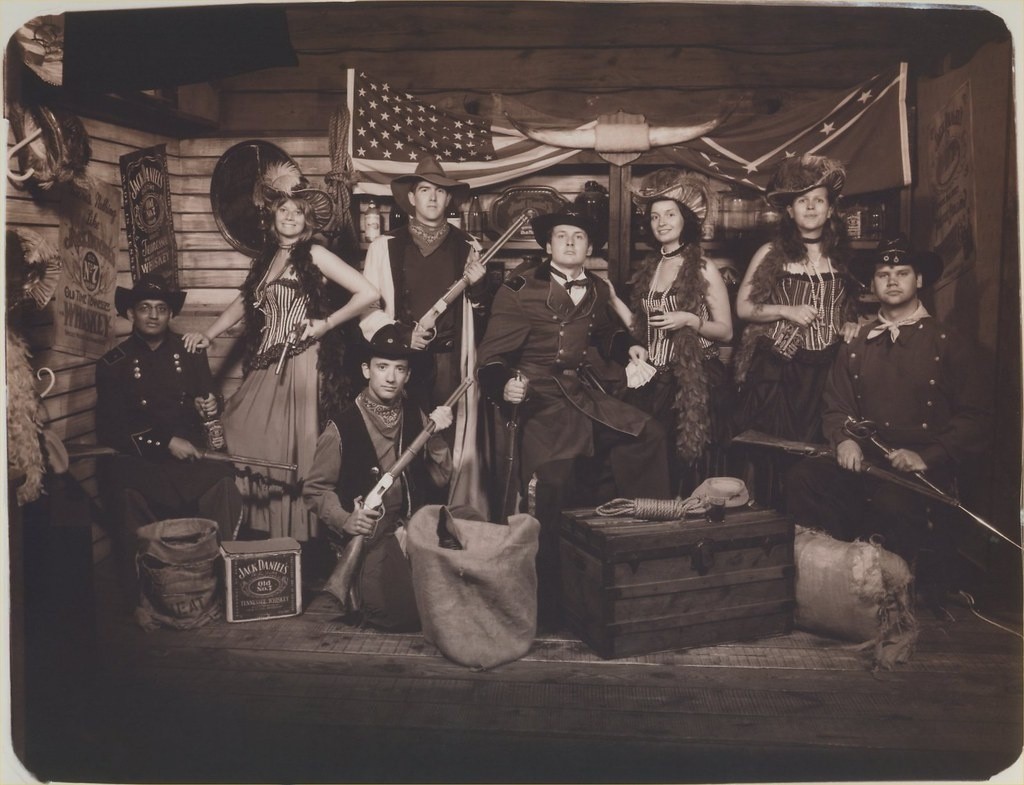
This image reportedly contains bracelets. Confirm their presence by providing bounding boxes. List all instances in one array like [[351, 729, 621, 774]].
[[695, 316, 703, 329]]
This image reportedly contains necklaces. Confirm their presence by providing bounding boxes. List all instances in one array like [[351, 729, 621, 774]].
[[255, 243, 298, 303], [646, 256, 685, 361], [660, 243, 687, 259], [280, 245, 294, 249], [802, 234, 824, 244]]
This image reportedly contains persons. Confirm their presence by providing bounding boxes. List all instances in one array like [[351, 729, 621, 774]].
[[301, 325, 455, 623], [475, 202, 674, 587], [360, 156, 496, 457], [736, 154, 868, 520], [600, 196, 735, 504], [181, 159, 382, 545], [95, 272, 241, 548], [821, 232, 993, 594]]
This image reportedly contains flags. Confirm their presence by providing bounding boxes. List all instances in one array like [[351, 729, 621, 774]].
[[346, 66, 597, 196]]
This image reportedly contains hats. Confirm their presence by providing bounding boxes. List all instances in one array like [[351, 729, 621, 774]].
[[360, 324, 421, 359], [765, 154, 847, 210], [633, 168, 718, 240], [872, 231, 917, 267], [531, 202, 602, 256], [252, 164, 336, 234], [390, 157, 470, 217], [114, 273, 188, 320]]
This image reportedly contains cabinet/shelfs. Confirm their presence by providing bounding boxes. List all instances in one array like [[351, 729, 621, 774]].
[[347, 146, 911, 278]]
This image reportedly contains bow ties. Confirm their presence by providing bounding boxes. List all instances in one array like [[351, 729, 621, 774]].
[[547, 268, 593, 291]]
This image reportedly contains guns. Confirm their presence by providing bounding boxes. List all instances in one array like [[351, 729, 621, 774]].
[[500, 368, 522, 531], [274, 323, 315, 375], [61, 439, 299, 471], [319, 376, 480, 606], [728, 426, 960, 509], [410, 201, 532, 343]]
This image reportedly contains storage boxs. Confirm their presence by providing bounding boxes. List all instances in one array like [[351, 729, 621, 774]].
[[555, 508, 798, 662], [219, 535, 302, 624]]
